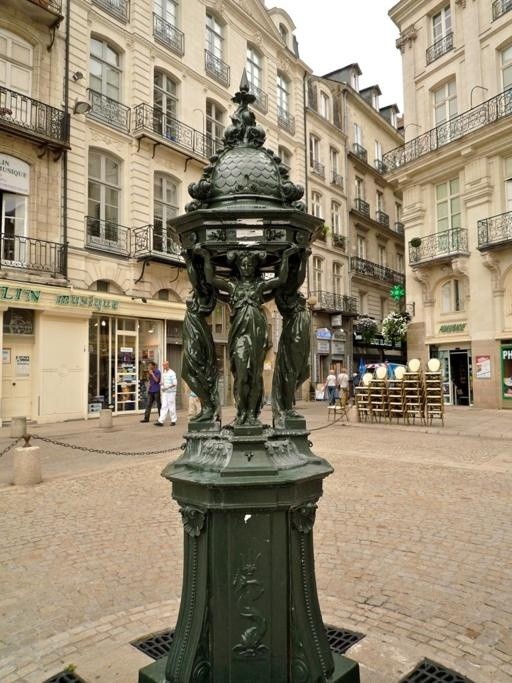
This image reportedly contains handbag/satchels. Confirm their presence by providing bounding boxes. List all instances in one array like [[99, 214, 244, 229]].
[[336, 383, 341, 392]]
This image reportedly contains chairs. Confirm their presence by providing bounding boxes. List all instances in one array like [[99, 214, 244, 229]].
[[328, 357, 445, 426]]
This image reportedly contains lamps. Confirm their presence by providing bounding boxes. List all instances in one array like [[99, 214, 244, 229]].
[[62, 93, 93, 115]]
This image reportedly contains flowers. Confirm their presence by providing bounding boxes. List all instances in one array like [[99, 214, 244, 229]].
[[380, 310, 410, 344], [353, 315, 380, 342]]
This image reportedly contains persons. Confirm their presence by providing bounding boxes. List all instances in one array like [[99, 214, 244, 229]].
[[180, 241, 310, 427], [139, 362, 160, 423], [321, 368, 357, 406], [152, 361, 178, 425]]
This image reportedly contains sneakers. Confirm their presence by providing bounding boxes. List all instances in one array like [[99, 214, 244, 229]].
[[168, 422, 176, 426], [153, 422, 163, 426], [139, 420, 150, 422]]
